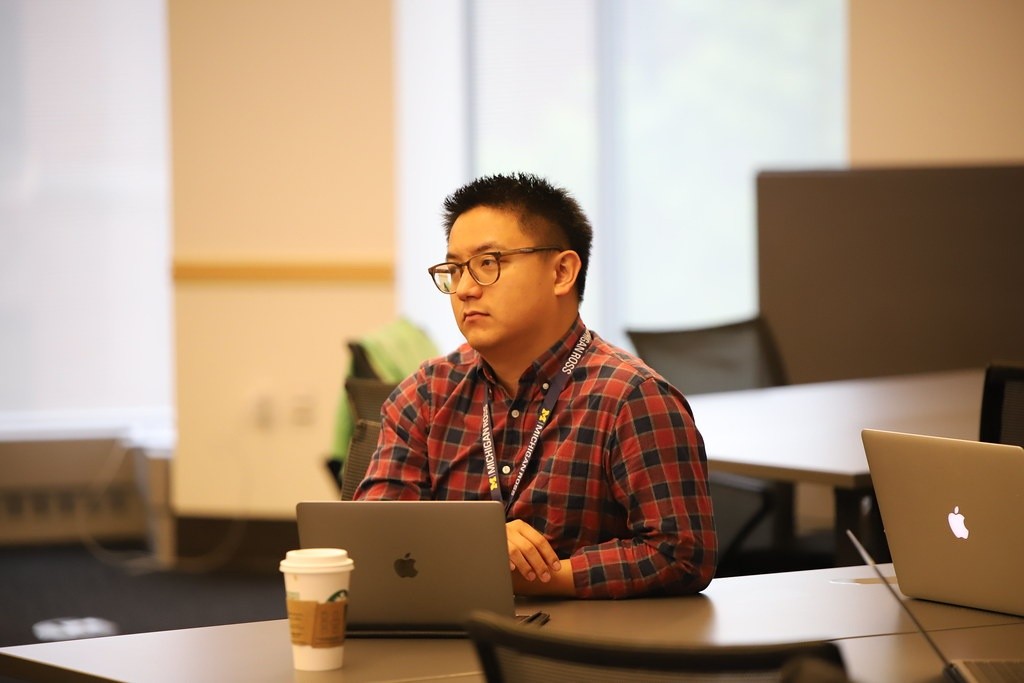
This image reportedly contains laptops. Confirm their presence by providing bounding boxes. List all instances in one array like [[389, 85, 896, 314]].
[[296, 501, 550, 637], [861, 428, 1024, 618]]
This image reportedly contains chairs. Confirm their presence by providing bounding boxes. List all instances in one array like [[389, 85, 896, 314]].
[[624, 312, 864, 562]]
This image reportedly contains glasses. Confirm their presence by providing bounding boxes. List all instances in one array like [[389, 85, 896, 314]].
[[428, 245, 565, 295]]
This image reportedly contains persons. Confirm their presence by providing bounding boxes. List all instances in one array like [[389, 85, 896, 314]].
[[350, 170, 720, 599]]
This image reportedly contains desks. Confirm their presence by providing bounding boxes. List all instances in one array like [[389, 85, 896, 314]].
[[672, 358, 1023, 566], [0, 548, 1023, 680]]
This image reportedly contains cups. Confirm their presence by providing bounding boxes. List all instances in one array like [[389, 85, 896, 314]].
[[279, 548, 355, 671]]
